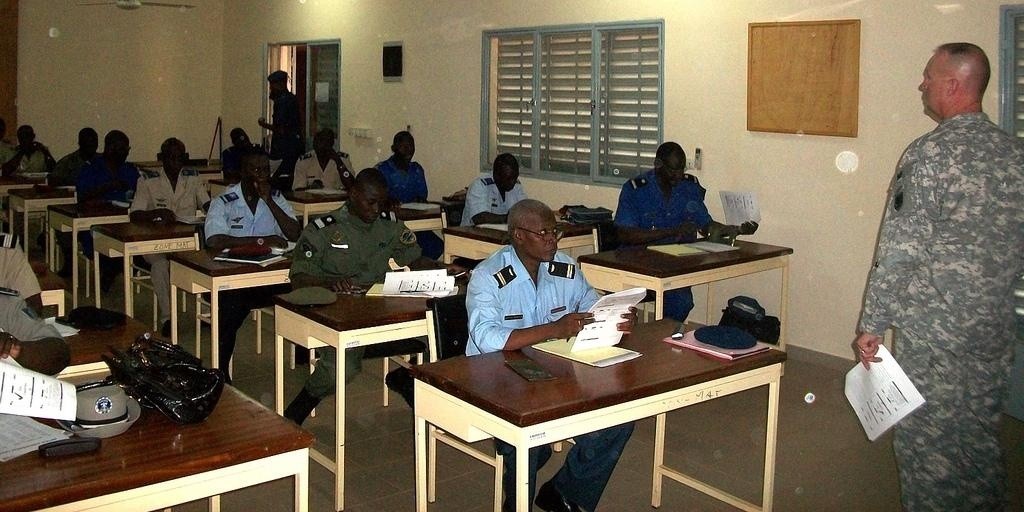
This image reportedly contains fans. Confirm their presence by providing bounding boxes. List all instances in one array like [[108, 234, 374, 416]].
[[77, 0, 197, 10]]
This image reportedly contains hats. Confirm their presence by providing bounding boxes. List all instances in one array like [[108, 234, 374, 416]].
[[267, 70, 287, 81], [56, 380, 142, 438], [278, 287, 338, 305], [229, 237, 272, 257], [694, 325, 756, 349]]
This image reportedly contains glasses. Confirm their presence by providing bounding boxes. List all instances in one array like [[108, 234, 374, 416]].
[[660, 162, 688, 176], [243, 167, 274, 177], [516, 226, 564, 241], [107, 147, 131, 152]]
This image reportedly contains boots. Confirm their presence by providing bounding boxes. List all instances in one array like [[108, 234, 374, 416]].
[[56, 238, 76, 278], [386, 355, 416, 407], [283, 387, 320, 424]]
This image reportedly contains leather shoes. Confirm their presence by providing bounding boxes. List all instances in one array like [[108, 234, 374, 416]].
[[296, 345, 320, 365], [218, 367, 231, 385], [534, 482, 581, 512], [162, 319, 178, 337], [99, 267, 115, 289], [503, 497, 532, 512]]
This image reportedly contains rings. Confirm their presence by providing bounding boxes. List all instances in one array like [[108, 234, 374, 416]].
[[578, 320, 581, 326], [861, 351, 865, 355]]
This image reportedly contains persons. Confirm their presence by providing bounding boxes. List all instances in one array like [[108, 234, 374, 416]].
[[458, 153, 526, 268], [0, 118, 140, 377], [851, 44, 1019, 510], [222, 70, 355, 194], [129, 138, 212, 338], [374, 131, 444, 263], [285, 168, 422, 430], [613, 142, 758, 323], [205, 148, 320, 387], [463, 199, 634, 512]]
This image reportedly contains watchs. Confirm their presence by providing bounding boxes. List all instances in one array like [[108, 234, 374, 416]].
[[257, 236, 264, 246]]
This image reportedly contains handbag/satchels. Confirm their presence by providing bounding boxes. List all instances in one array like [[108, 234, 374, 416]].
[[717, 295, 780, 344], [101, 335, 224, 424], [557, 204, 614, 225]]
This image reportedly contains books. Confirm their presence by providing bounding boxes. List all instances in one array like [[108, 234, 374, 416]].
[[662, 330, 770, 361]]
